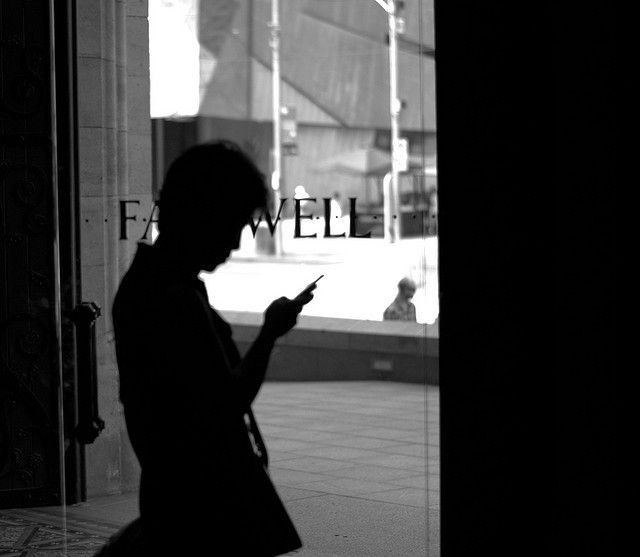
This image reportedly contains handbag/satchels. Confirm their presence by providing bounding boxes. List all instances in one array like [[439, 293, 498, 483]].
[[148, 457, 302, 556]]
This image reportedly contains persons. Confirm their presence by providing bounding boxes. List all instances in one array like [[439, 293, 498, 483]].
[[384, 277, 416, 322], [112, 138, 315, 557]]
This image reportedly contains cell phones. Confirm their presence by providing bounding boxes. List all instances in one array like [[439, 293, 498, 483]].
[[292, 275, 325, 298]]
